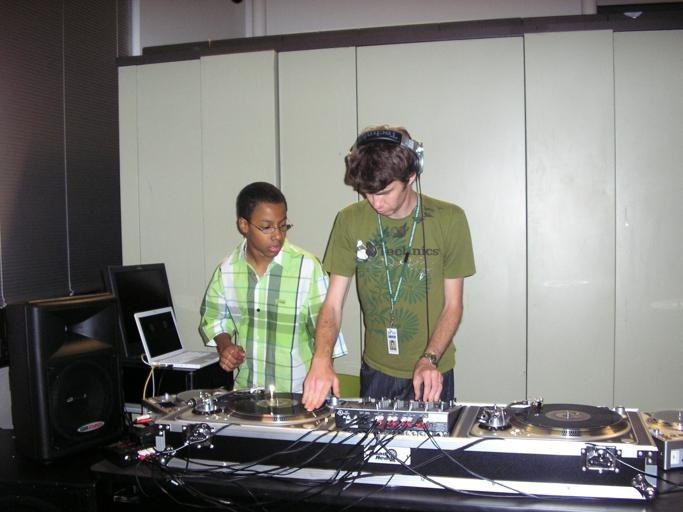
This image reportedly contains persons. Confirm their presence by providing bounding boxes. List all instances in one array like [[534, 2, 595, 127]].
[[301, 124, 467, 412], [207, 182, 335, 394]]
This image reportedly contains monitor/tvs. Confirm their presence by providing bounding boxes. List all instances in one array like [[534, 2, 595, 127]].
[[103, 263, 176, 359]]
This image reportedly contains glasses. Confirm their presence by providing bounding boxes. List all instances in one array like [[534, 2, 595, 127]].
[[243, 217, 294, 233]]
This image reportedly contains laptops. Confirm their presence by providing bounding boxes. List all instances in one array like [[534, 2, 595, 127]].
[[133, 306, 220, 369]]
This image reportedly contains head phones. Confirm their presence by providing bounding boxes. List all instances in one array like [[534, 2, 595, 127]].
[[348, 130, 425, 174]]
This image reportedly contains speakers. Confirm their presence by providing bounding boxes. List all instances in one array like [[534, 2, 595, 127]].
[[4, 292, 123, 466]]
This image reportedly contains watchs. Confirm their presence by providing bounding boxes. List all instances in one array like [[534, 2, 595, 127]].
[[419, 353, 440, 368]]
